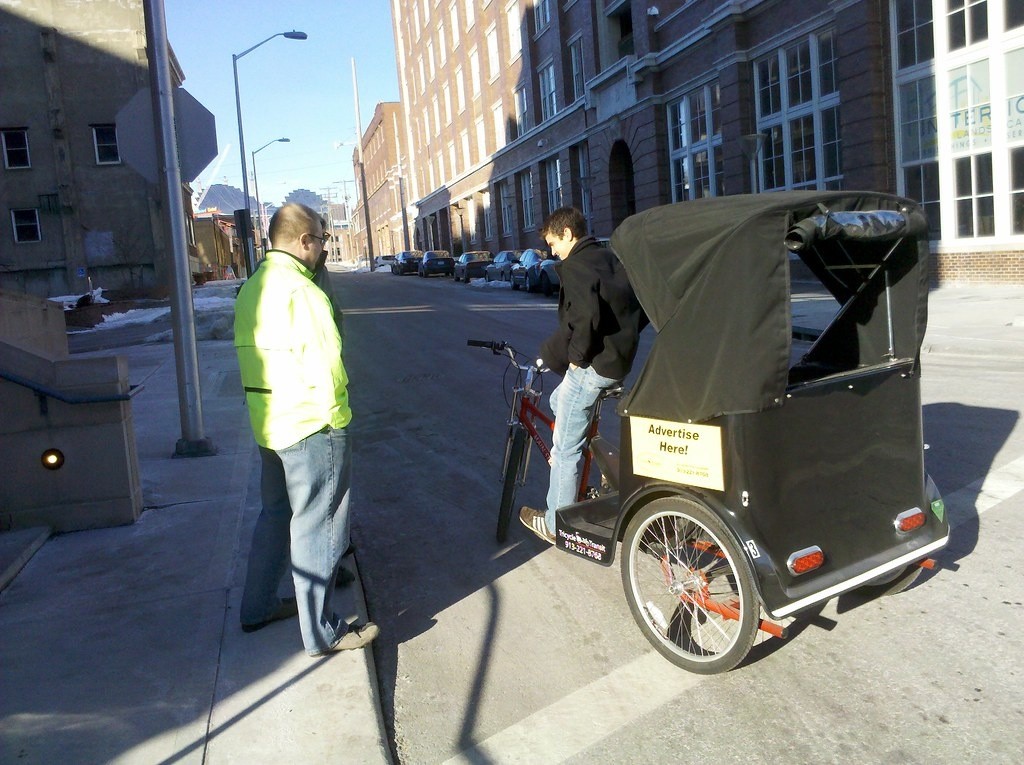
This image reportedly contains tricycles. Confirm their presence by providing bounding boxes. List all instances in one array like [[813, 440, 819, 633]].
[[464, 190, 951, 677]]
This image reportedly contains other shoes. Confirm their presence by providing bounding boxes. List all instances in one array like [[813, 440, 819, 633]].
[[342, 541, 356, 557], [333, 564, 356, 586]]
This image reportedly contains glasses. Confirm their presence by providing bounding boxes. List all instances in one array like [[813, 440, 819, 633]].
[[307, 233, 326, 246], [323, 231, 331, 240]]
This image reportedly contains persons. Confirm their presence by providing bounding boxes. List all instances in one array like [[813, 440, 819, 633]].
[[233, 202, 381, 658], [517, 205, 650, 546]]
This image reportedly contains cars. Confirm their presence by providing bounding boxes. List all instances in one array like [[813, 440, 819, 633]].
[[509, 248, 558, 293], [485, 250, 525, 282], [539, 238, 611, 297], [391, 251, 425, 275], [375, 255, 395, 269], [418, 250, 455, 278], [357, 258, 370, 269], [453, 251, 495, 284]]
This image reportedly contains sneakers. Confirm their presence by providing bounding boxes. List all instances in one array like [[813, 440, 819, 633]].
[[241, 597, 298, 633], [518, 506, 558, 545], [309, 620, 382, 657]]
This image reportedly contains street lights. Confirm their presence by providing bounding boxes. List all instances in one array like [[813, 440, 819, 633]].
[[232, 29, 308, 275], [251, 138, 291, 259]]
